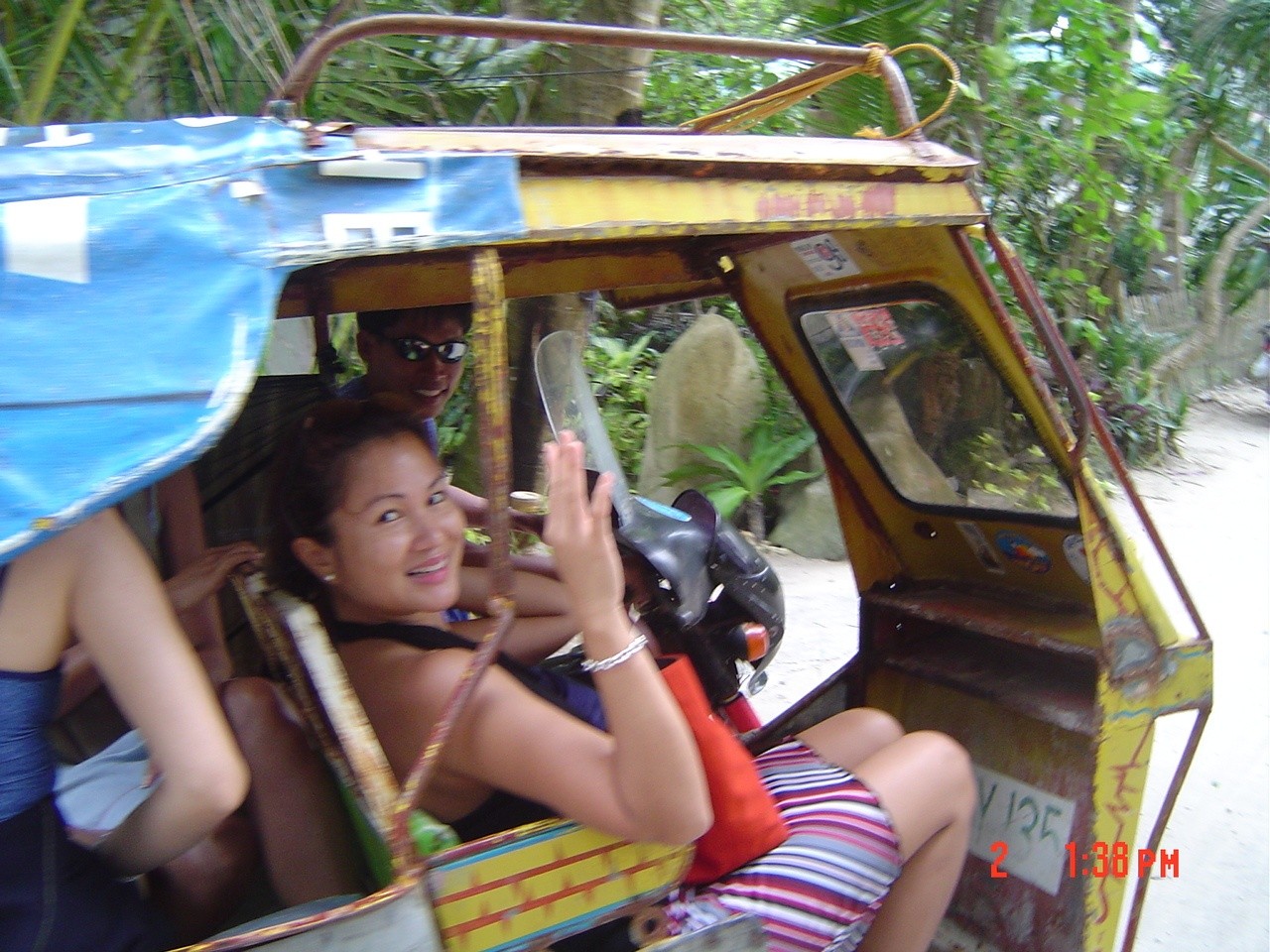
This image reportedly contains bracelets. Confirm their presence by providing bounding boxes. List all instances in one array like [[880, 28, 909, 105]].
[[579, 634, 649, 673]]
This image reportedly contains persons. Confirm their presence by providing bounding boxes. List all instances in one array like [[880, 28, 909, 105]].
[[260, 305, 975, 952], [0, 505, 252, 952], [53, 468, 368, 926]]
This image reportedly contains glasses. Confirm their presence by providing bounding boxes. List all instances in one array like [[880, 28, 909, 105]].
[[376, 333, 468, 363]]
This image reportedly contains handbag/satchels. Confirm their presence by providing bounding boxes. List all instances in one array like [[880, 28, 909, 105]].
[[650, 652, 794, 888]]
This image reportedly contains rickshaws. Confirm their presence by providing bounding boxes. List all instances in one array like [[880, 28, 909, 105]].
[[0, 12, 1219, 952]]
